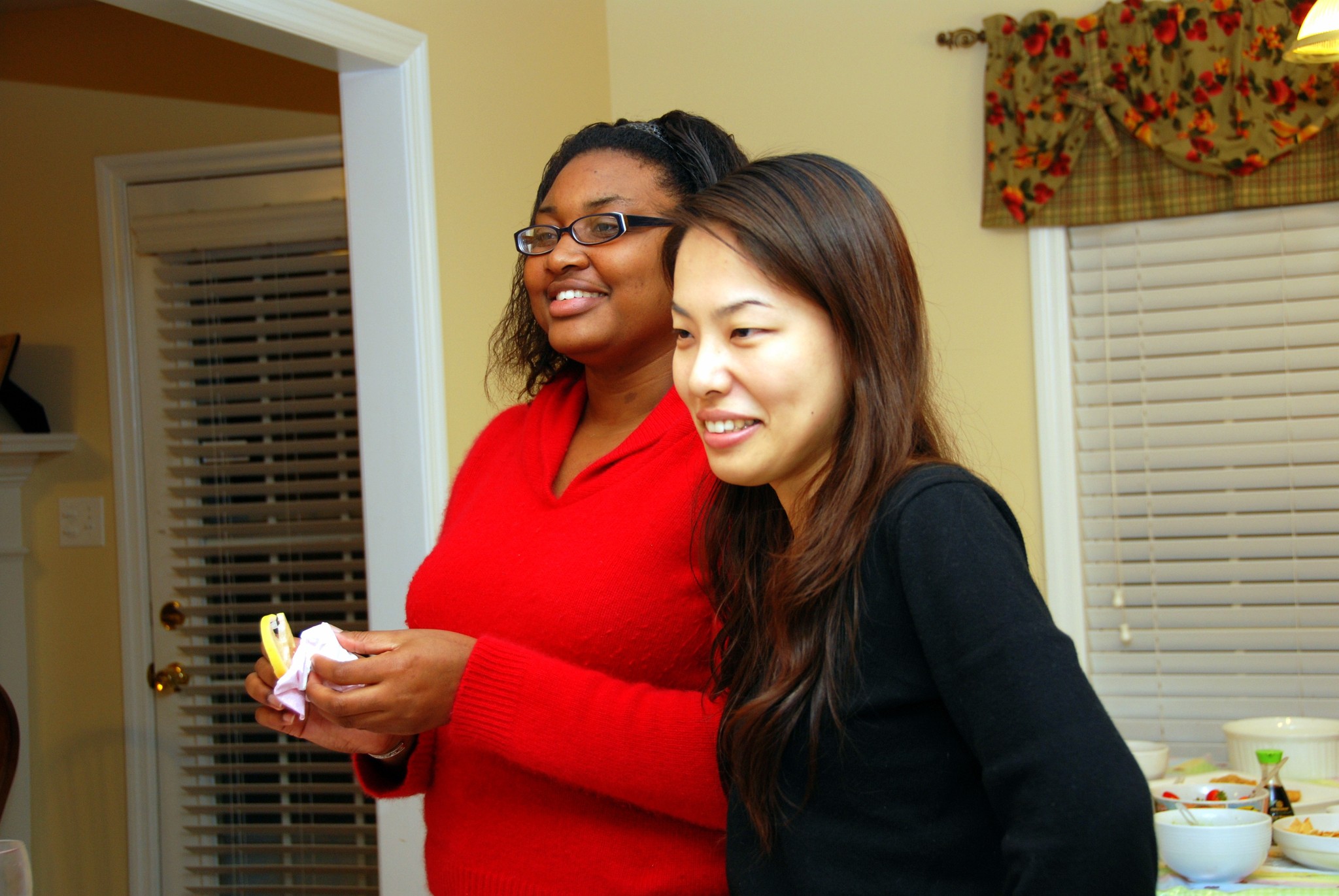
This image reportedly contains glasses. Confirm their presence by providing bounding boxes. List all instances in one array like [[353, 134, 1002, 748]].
[[514, 212, 675, 255]]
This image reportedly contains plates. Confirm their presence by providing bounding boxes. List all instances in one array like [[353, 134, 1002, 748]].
[[1148, 769, 1339, 813]]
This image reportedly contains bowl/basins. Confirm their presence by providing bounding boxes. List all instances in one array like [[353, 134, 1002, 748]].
[[1272, 814, 1339, 873], [1151, 783, 1270, 813], [1126, 739, 1169, 780], [1220, 716, 1339, 780], [1153, 808, 1273, 883]]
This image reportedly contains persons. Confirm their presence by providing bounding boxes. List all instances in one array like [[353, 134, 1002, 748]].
[[659, 153, 1157, 896], [244, 109, 752, 896]]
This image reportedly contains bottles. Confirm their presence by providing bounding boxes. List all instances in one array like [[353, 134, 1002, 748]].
[[1254, 750, 1296, 858]]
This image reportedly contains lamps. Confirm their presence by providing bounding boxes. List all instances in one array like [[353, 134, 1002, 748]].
[[1284, 0, 1339, 64]]
